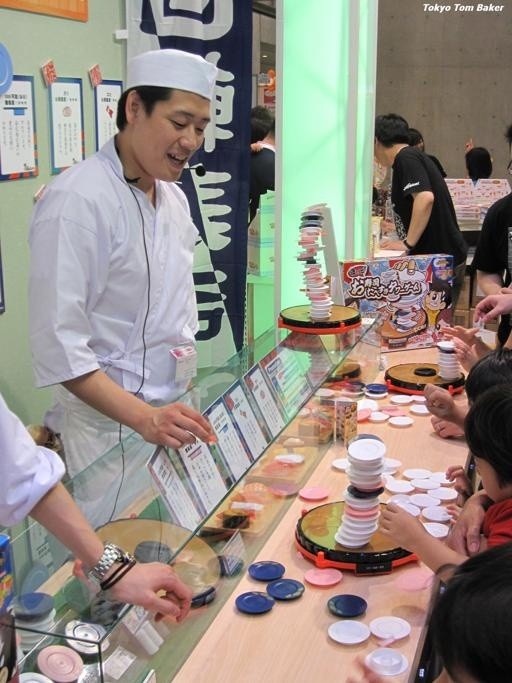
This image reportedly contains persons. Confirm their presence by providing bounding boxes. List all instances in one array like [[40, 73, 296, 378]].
[[250, 105, 275, 221], [427, 414, 468, 441], [377, 382, 512, 583], [472, 124, 512, 345], [420, 348, 512, 428], [464, 146, 493, 186], [25, 48, 219, 531], [375, 114, 469, 281], [422, 281, 455, 336], [425, 540, 512, 683], [444, 486, 494, 558], [408, 128, 447, 181], [0, 392, 191, 624]]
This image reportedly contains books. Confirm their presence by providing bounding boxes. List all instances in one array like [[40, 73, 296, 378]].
[[258, 330, 334, 422], [221, 378, 268, 462], [175, 432, 228, 519], [241, 364, 287, 441], [0, 75, 42, 181], [50, 77, 85, 176], [145, 441, 203, 542], [95, 80, 125, 156], [200, 396, 254, 485]]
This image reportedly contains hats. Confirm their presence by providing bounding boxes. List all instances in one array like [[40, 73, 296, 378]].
[[124, 47, 219, 102]]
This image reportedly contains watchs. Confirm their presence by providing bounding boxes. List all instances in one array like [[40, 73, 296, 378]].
[[401, 237, 414, 253], [86, 540, 133, 581]]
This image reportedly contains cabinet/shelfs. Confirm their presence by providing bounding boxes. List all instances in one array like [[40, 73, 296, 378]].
[[1, 313, 494, 682]]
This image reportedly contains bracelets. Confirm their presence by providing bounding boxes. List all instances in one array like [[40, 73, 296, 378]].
[[99, 561, 138, 592]]
